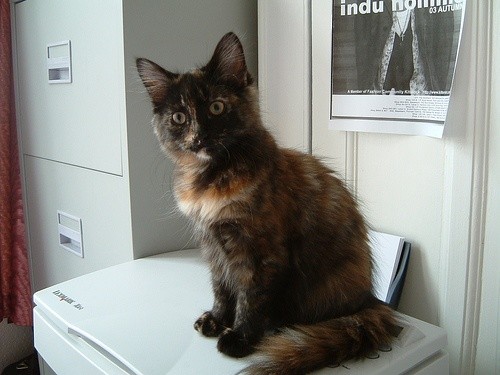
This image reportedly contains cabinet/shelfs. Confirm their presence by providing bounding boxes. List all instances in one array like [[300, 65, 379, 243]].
[[9, 0, 256, 295]]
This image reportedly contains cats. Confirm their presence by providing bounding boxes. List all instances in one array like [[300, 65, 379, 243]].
[[125, 31, 403, 375]]
